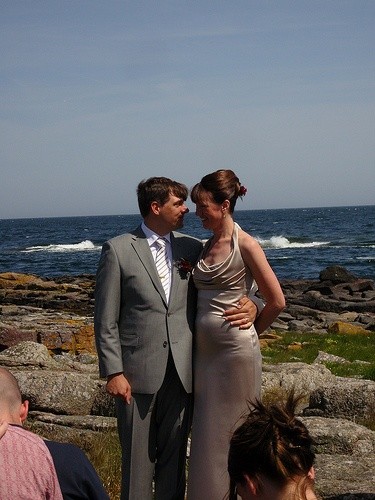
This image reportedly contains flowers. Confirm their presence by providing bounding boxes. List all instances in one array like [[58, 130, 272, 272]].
[[175, 256, 191, 280]]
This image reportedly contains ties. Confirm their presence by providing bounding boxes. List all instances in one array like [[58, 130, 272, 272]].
[[155, 238, 170, 307]]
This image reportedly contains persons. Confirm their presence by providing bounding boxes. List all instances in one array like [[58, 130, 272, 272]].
[[0, 367, 111, 500], [227, 389, 375, 500], [94, 177, 264, 500], [187, 169, 285, 500]]
